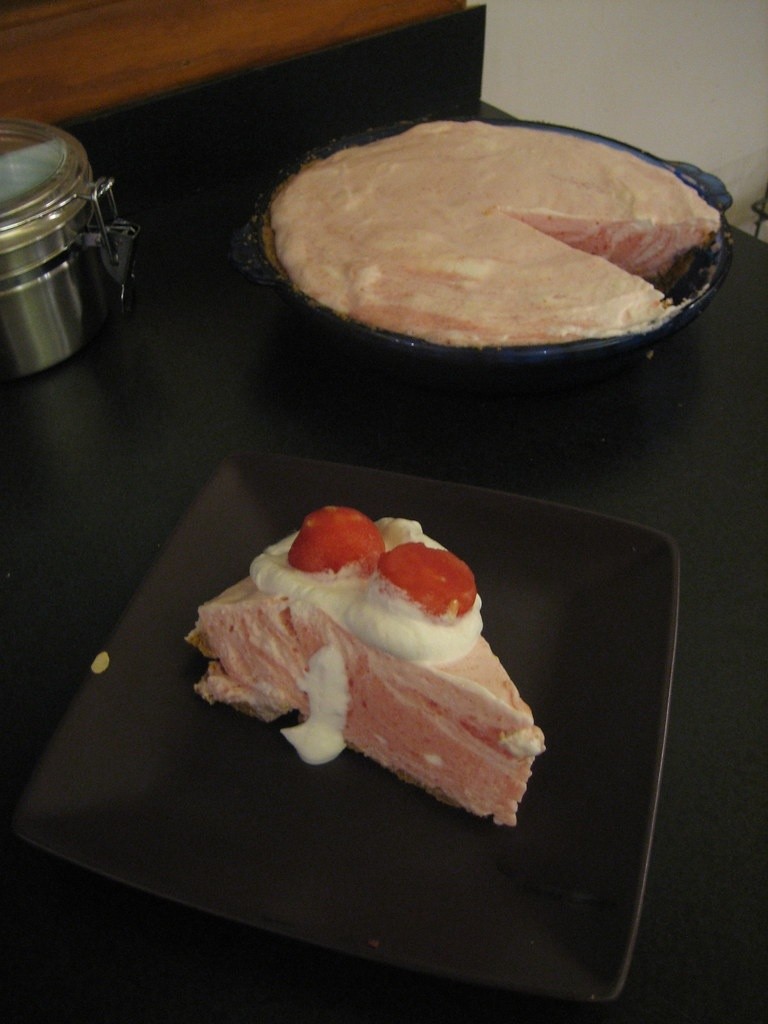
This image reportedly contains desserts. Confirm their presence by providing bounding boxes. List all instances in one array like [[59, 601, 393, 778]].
[[184, 504, 547, 830], [266, 118, 720, 349]]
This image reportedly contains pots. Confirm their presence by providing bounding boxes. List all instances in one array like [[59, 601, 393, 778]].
[[0, 117, 138, 377]]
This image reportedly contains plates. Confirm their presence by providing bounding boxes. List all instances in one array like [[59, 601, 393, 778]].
[[11, 448, 681, 1005], [230, 109, 734, 388]]
